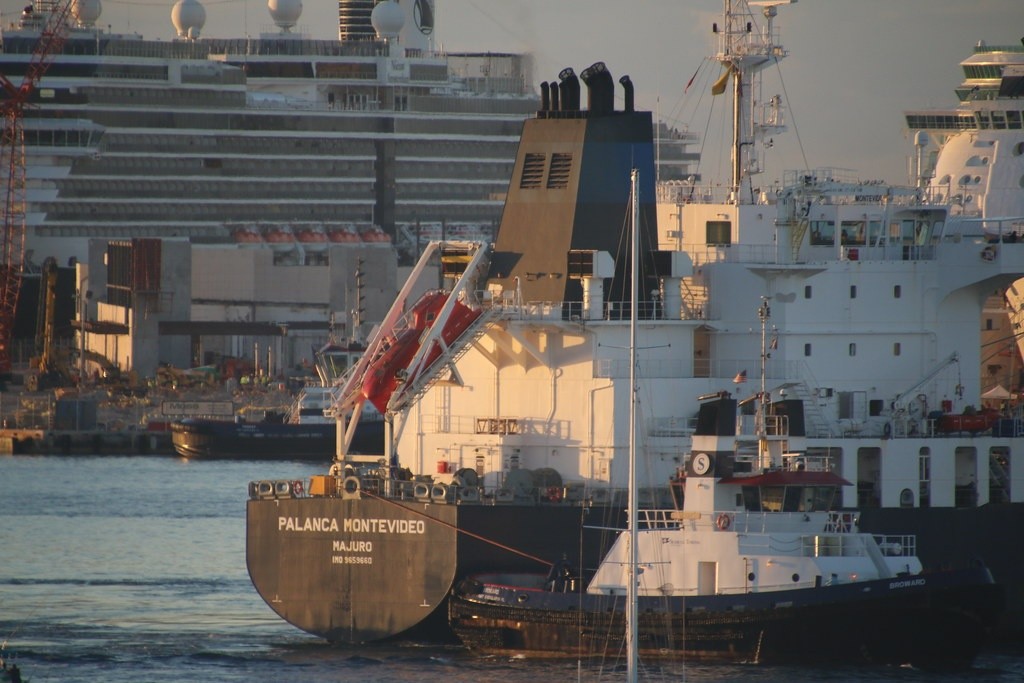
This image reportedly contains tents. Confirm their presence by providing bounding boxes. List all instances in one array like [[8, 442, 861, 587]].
[[981, 385, 1017, 409]]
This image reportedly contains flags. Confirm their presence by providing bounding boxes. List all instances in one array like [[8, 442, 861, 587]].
[[733, 370, 747, 383], [712, 63, 732, 95]]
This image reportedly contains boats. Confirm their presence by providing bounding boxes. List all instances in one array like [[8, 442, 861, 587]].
[[447, 295, 1004, 667], [172, 256, 393, 459], [244, 0, 1024, 644]]
